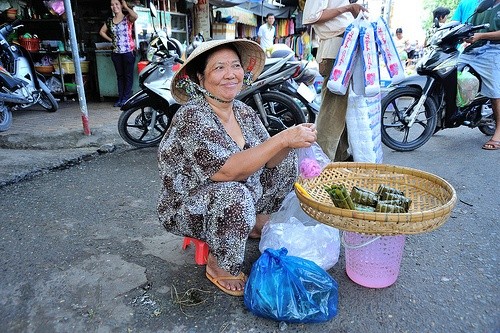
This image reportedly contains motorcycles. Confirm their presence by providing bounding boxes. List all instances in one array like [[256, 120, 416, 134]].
[[0, 17, 59, 133], [117, 1, 321, 149], [381, 0, 497, 152]]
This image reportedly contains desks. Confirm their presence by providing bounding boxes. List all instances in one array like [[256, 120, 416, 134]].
[[96, 49, 143, 102]]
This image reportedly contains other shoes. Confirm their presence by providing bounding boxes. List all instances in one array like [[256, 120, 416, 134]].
[[113, 100, 126, 107]]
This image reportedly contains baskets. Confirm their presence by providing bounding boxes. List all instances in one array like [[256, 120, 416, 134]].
[[294, 161, 458, 235], [18, 37, 39, 53], [34, 66, 54, 74], [52, 60, 90, 74], [64, 83, 79, 92]]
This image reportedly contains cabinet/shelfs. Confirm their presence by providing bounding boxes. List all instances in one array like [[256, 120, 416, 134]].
[[133, 8, 189, 50], [17, 18, 87, 101]]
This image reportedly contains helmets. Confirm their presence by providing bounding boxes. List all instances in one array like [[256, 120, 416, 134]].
[[166, 38, 184, 58]]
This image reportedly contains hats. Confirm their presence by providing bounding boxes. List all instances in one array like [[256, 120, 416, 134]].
[[170, 38, 266, 105]]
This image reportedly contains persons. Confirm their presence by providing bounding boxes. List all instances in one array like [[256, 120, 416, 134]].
[[392, 28, 411, 64], [302, 0, 368, 162], [157, 38, 319, 297], [424, 7, 450, 49], [258, 14, 276, 53], [99, 0, 138, 107], [451, 0, 500, 150]]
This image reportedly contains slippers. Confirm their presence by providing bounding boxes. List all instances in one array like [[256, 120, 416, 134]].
[[482, 139, 500, 150], [250, 232, 261, 239], [205, 268, 249, 297]]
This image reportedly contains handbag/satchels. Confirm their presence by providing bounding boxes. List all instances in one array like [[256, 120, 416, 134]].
[[241, 248, 341, 324]]
